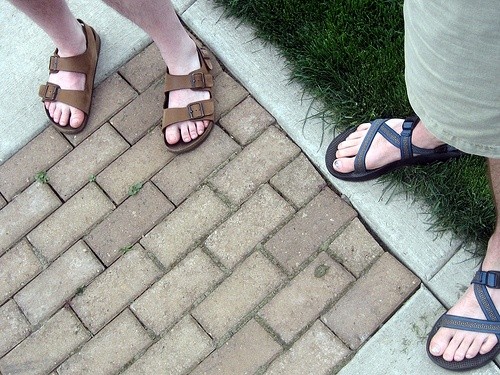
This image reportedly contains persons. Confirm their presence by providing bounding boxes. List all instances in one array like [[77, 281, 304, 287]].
[[5, 0, 212, 156], [324, 0, 500, 373]]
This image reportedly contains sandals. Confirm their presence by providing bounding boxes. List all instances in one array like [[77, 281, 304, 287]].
[[39, 19, 102, 136], [162, 44, 215, 154], [325, 115, 462, 181], [426, 270, 500, 371]]
